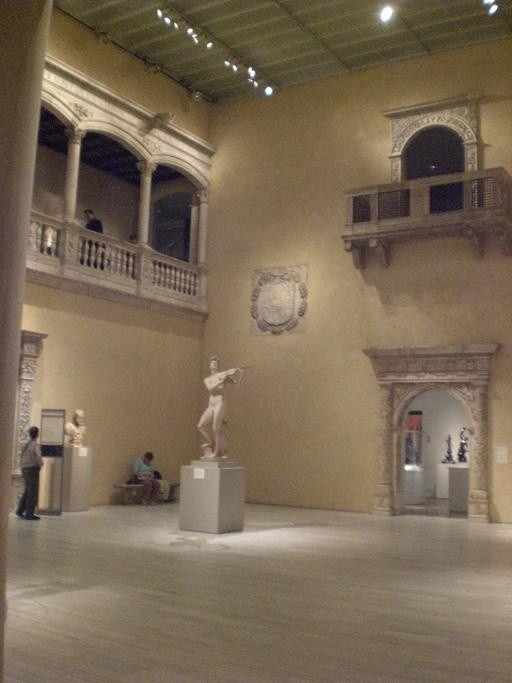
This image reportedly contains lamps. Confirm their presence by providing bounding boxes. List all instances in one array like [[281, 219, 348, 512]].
[[157, 7, 215, 49], [225, 52, 278, 95]]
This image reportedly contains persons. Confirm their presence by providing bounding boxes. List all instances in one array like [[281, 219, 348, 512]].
[[14, 426, 43, 520], [125, 233, 137, 280], [63, 409, 86, 447], [81, 208, 106, 271], [405, 432, 417, 465], [132, 451, 162, 505], [196, 356, 245, 458]]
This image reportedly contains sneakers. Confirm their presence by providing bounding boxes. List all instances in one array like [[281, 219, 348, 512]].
[[15, 512, 40, 520]]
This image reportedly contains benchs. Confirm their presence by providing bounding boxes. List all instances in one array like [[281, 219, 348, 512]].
[[115, 482, 180, 506]]
